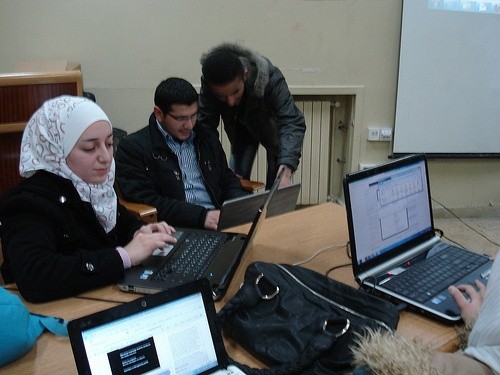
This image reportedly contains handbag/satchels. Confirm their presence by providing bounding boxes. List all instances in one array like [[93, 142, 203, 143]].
[[217, 261, 399, 375]]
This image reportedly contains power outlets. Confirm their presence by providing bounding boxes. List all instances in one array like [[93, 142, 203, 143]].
[[367, 126, 392, 142]]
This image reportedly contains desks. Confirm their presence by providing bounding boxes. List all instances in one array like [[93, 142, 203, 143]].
[[1, 202, 467, 375]]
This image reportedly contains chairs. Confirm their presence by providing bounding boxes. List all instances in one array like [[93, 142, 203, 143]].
[[113, 148, 265, 224]]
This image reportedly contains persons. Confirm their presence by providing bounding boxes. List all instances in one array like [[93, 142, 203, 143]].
[[114, 78, 255, 231], [350, 246, 500, 375], [198, 43, 306, 191], [0, 95, 178, 306]]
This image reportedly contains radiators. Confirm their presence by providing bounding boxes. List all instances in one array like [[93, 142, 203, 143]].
[[217, 101, 340, 209]]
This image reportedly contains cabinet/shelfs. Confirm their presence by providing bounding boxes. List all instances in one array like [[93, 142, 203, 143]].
[[0, 70, 84, 289]]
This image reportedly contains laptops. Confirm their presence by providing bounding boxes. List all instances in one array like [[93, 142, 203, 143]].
[[67, 278, 249, 375], [344, 153, 495, 325], [217, 183, 300, 232], [115, 167, 285, 300]]
